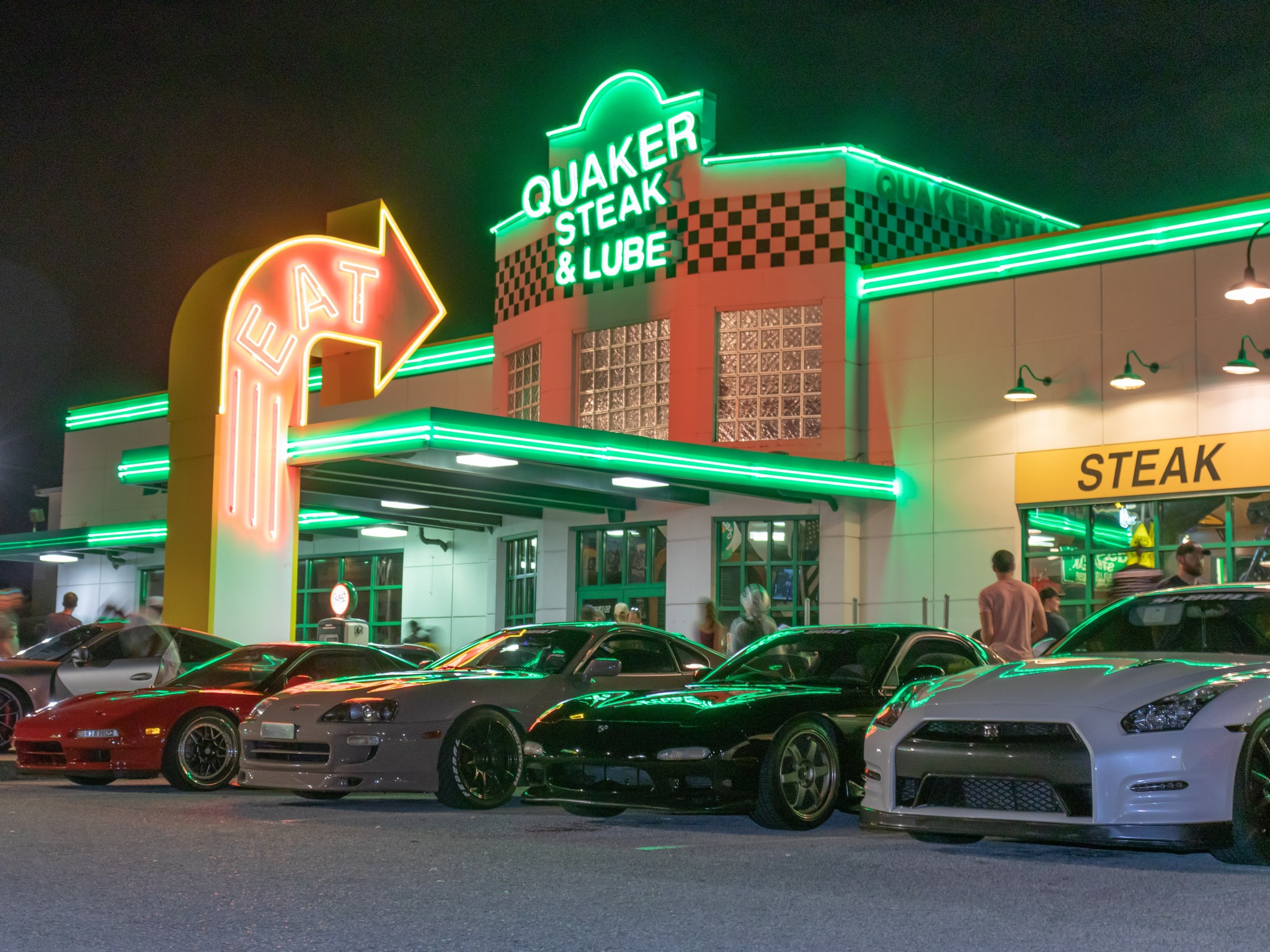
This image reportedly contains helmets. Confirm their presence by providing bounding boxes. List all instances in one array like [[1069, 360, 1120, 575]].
[[739, 583, 771, 623]]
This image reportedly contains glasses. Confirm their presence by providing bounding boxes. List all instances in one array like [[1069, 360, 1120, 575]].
[[630, 607, 639, 612]]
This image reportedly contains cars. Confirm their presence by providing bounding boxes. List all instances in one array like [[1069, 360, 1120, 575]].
[[860, 579, 1270, 869], [520, 621, 1008, 831], [0, 620, 249, 753], [238, 620, 735, 811], [11, 641, 424, 791]]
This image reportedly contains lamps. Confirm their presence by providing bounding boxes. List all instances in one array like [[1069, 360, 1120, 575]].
[[1221, 334, 1270, 374], [1108, 349, 1161, 390], [1003, 365, 1052, 404], [29, 507, 45, 533]]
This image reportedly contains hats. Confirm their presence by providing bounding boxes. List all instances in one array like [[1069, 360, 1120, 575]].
[[614, 602, 629, 616], [1176, 541, 1211, 557], [1039, 587, 1066, 600]]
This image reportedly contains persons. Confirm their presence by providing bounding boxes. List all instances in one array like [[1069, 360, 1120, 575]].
[[1032, 586, 1071, 642], [1143, 541, 1211, 649], [980, 549, 1048, 663], [694, 598, 723, 653], [0, 621, 19, 657], [41, 592, 82, 640], [582, 603, 647, 653], [726, 583, 787, 656]]
